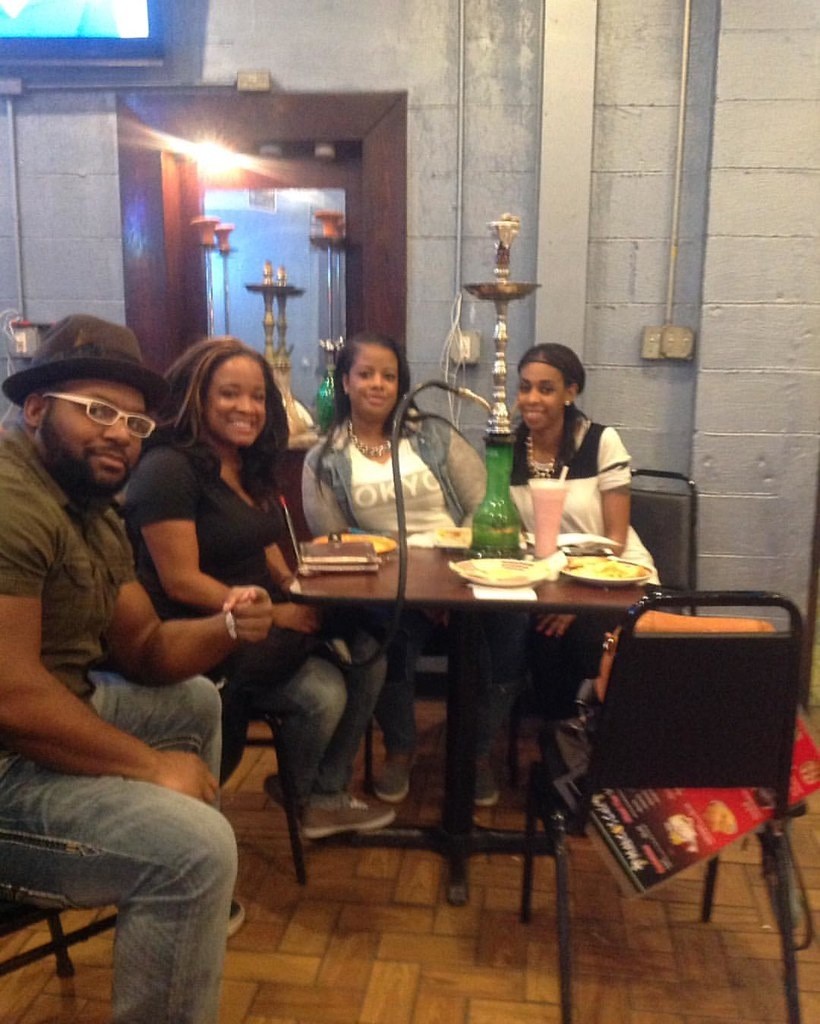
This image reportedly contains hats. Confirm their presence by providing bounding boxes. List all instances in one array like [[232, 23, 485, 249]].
[[1, 313, 170, 407]]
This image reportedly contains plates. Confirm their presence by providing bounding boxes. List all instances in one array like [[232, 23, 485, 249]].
[[524, 530, 622, 550], [436, 528, 525, 547], [559, 557, 655, 586], [314, 535, 397, 554], [453, 558, 550, 586]]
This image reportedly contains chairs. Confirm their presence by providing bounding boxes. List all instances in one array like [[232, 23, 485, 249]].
[[515, 467, 697, 804], [517, 589, 810, 1024], [0, 890, 121, 980]]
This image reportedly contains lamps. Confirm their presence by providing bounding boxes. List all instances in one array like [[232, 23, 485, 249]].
[[236, 69, 271, 93]]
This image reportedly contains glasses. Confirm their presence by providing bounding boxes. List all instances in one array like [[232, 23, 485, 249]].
[[41, 389, 155, 438]]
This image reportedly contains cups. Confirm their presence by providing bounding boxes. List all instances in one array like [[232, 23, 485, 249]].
[[527, 478, 570, 559]]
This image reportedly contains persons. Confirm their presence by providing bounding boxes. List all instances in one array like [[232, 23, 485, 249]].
[[491, 341, 632, 792], [114, 336, 398, 839], [0, 313, 277, 1024], [302, 333, 519, 806], [308, 365, 329, 419]]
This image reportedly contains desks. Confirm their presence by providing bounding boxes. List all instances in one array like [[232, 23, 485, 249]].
[[286, 540, 653, 891]]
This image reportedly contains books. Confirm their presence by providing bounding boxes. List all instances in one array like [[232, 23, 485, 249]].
[[558, 700, 819, 897], [298, 540, 380, 572]]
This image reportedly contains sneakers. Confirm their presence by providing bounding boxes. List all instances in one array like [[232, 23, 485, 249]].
[[300, 789, 400, 838], [264, 772, 291, 805], [373, 747, 413, 803]]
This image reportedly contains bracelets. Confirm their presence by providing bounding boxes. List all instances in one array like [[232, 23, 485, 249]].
[[225, 612, 238, 639]]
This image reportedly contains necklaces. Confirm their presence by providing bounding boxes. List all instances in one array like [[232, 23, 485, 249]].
[[524, 429, 556, 478], [348, 420, 392, 457]]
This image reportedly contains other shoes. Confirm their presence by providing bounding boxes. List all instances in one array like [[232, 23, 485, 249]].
[[473, 753, 501, 806], [226, 900, 244, 940]]
